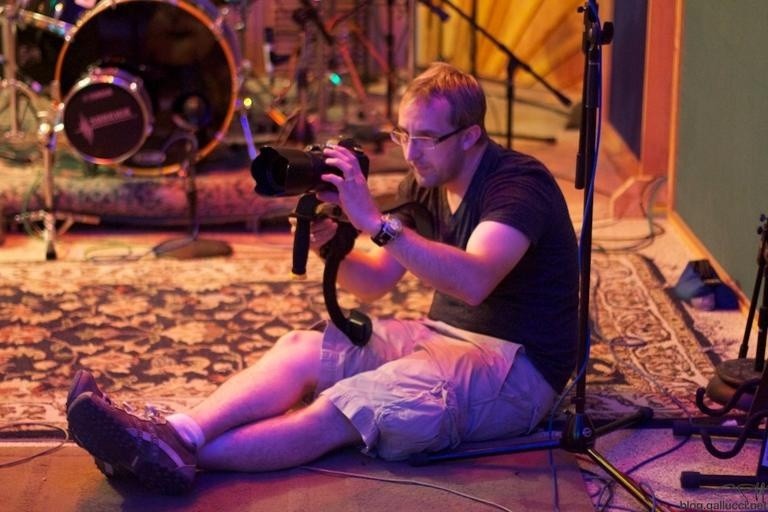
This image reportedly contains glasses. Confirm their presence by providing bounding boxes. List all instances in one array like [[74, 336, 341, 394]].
[[390, 127, 462, 150]]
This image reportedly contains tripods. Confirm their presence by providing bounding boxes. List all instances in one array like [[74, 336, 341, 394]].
[[405, 2, 671, 512]]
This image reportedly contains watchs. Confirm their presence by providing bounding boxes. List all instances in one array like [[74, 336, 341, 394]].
[[373, 213, 405, 249]]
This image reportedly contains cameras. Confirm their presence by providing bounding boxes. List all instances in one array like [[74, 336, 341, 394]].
[[250, 135, 376, 223]]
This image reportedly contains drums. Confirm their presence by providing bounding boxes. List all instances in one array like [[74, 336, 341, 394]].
[[56, 0, 253, 177], [54, 65, 152, 167]]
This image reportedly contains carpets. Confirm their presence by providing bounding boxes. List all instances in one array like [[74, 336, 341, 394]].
[[0, 236, 747, 440]]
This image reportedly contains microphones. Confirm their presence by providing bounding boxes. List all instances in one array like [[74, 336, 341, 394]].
[[262, 26, 279, 74]]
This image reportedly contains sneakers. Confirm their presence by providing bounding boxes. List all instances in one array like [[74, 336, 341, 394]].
[[67, 391, 207, 497], [66, 370, 134, 481]]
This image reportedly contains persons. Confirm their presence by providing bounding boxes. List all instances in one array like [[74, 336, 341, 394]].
[[65, 61, 578, 499]]
